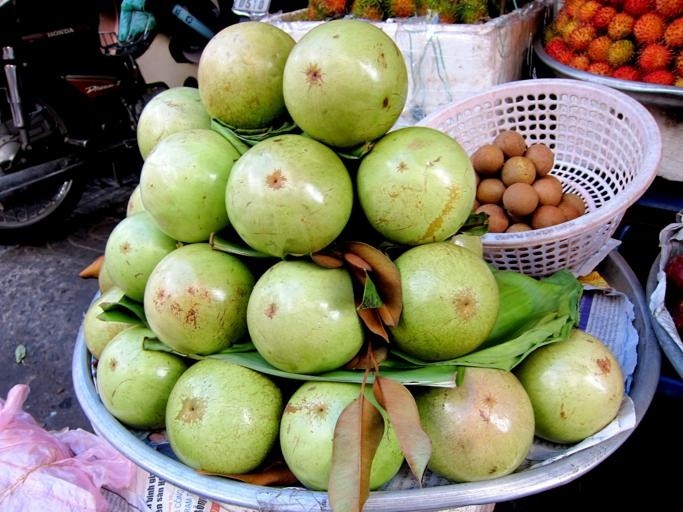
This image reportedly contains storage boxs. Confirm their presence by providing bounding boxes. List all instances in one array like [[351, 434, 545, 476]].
[[259, 0, 556, 135]]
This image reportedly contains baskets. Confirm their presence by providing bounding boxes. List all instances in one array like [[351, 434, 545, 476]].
[[417, 77, 663, 275]]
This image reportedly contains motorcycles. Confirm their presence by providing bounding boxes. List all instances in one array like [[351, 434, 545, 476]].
[[0, 30, 200, 245]]
